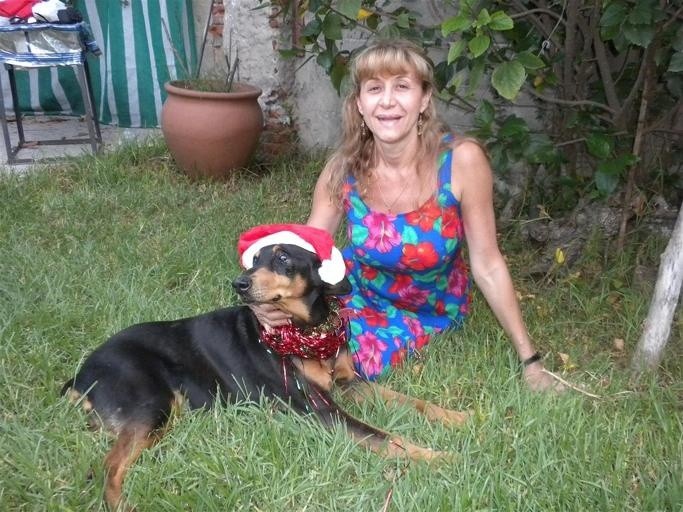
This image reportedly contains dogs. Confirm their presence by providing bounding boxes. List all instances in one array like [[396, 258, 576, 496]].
[[61, 224, 476, 512]]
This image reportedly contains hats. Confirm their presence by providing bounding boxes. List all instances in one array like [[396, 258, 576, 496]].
[[237, 223, 347, 285]]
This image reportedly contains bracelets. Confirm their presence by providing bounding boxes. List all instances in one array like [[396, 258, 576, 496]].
[[520, 351, 540, 366]]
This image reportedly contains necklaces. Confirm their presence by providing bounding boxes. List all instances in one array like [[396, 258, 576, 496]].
[[365, 160, 419, 214]]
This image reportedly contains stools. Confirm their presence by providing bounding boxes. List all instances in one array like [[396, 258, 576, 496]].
[[0, 22, 107, 166]]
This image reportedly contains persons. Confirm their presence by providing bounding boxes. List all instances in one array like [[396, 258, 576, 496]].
[[249, 41, 562, 389]]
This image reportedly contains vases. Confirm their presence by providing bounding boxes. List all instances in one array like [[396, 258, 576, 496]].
[[160, 79, 263, 183]]
